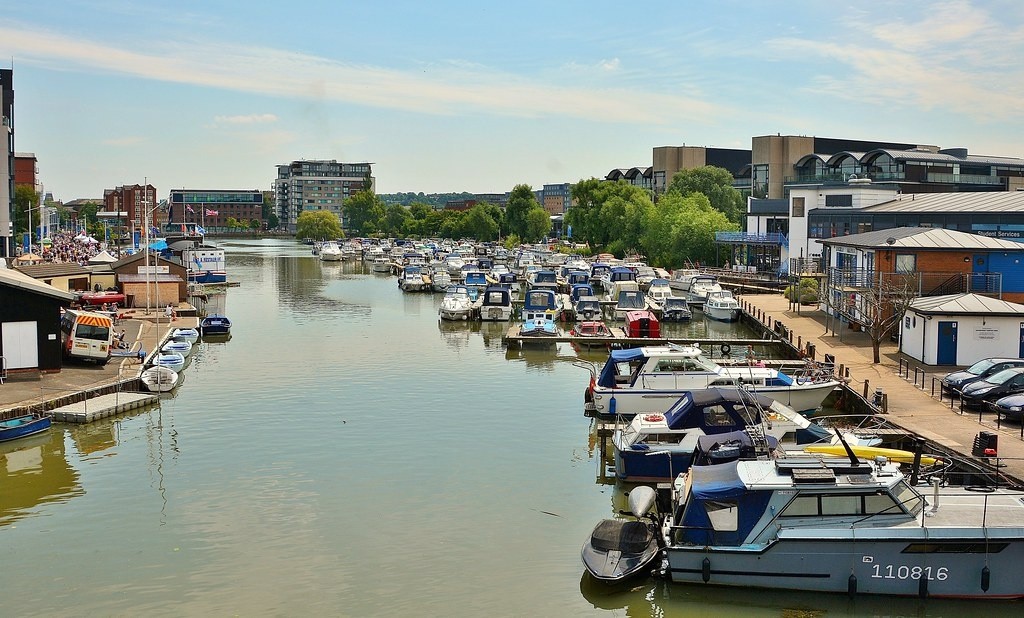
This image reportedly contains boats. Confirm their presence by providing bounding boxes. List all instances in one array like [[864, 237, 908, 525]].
[[606, 385, 883, 483], [142, 365, 180, 393], [580, 516, 658, 587], [201, 315, 233, 335], [152, 350, 184, 373], [162, 340, 192, 359], [297, 231, 742, 348], [580, 346, 841, 422], [665, 456, 1024, 602], [173, 327, 200, 344]]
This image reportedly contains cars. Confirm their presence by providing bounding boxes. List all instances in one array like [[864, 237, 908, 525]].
[[961, 367, 1024, 412], [942, 357, 1024, 398], [993, 392, 1024, 425]]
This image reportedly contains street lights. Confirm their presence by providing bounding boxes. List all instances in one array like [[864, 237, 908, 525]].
[[24, 200, 46, 266]]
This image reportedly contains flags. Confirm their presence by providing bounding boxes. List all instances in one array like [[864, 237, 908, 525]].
[[166, 203, 174, 229], [206, 209, 220, 217], [182, 224, 206, 236], [152, 227, 160, 234], [186, 204, 194, 214], [193, 258, 202, 268]]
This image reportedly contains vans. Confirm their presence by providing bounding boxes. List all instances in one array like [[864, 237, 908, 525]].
[[59, 308, 115, 367]]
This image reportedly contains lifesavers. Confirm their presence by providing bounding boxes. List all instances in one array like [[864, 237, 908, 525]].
[[644, 414, 663, 422], [720, 343, 731, 354], [811, 369, 824, 383]]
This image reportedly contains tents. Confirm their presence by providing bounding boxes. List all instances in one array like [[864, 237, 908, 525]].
[[17, 253, 44, 265], [36, 238, 52, 248], [80, 236, 99, 243], [88, 250, 118, 266], [74, 233, 87, 240]]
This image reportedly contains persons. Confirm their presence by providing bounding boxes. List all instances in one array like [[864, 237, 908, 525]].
[[744, 345, 755, 358], [166, 304, 177, 321], [33, 233, 155, 266], [167, 302, 173, 308], [101, 303, 118, 316], [117, 329, 125, 338], [112, 325, 119, 349], [724, 259, 730, 269]]
